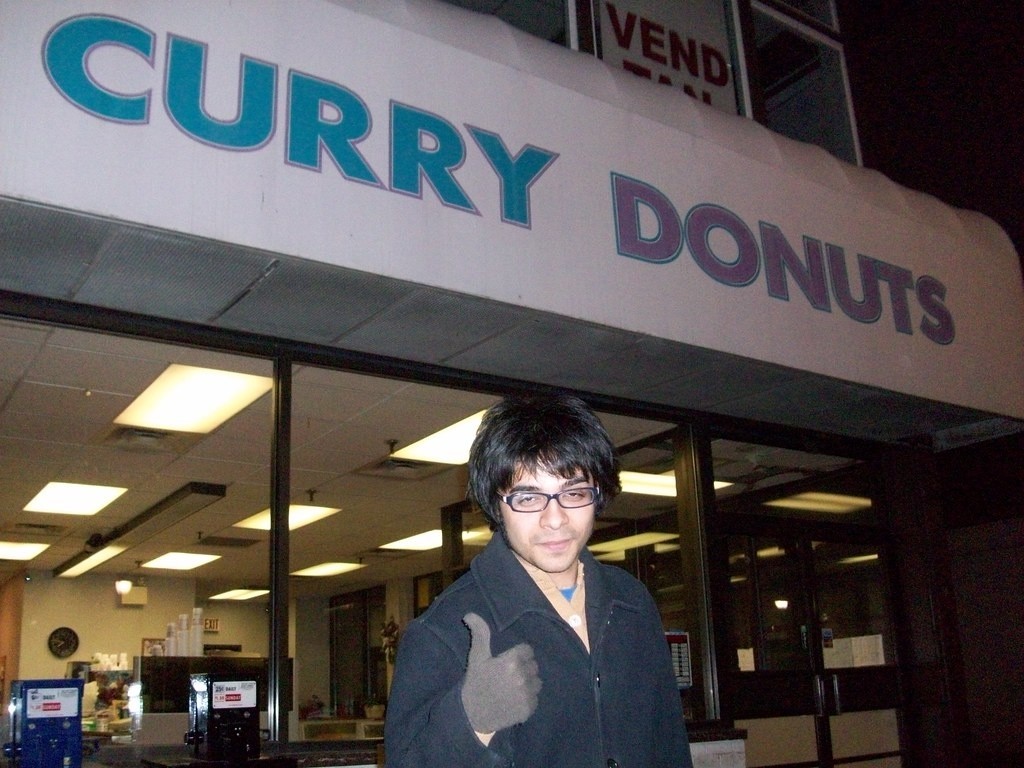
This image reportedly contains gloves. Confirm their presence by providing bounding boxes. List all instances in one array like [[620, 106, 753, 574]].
[[462, 612, 542, 734]]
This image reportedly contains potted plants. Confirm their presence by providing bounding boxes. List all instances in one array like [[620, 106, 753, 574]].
[[361, 693, 386, 719]]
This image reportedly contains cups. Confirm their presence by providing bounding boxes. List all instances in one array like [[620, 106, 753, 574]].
[[152, 608, 205, 656], [90, 653, 128, 670]]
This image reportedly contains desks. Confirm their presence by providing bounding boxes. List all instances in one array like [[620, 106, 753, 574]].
[[300, 717, 386, 741]]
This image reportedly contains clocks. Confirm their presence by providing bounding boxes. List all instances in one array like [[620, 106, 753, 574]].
[[48, 627, 79, 659]]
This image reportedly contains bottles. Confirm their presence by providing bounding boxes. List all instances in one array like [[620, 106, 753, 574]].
[[822, 614, 833, 648], [96, 714, 110, 732]]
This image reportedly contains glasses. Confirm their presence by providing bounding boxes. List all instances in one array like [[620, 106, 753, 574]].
[[496, 481, 599, 512]]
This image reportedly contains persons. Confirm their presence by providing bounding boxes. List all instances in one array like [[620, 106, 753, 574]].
[[95, 672, 133, 709], [382, 394, 692, 767]]
